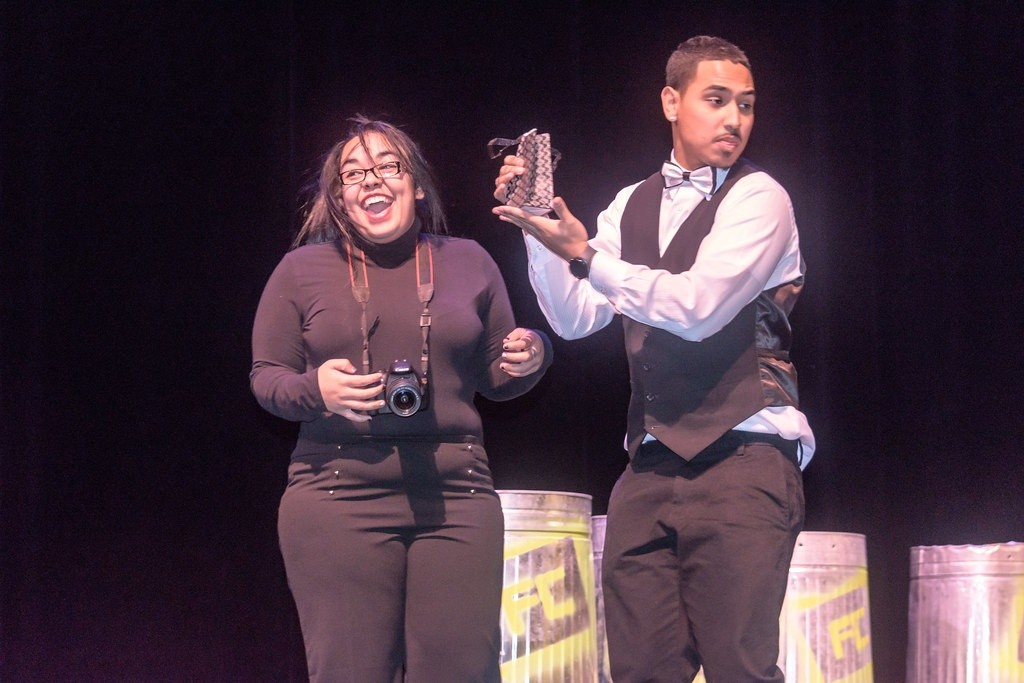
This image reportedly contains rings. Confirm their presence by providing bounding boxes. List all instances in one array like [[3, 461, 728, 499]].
[[521, 335, 533, 350], [532, 346, 540, 353]]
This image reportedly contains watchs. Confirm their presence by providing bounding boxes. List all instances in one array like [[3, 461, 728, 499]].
[[568, 246, 599, 280]]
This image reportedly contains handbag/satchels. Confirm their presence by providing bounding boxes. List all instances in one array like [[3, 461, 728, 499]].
[[487, 129, 562, 217]]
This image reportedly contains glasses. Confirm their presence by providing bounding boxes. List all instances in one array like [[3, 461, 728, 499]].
[[339, 161, 407, 186]]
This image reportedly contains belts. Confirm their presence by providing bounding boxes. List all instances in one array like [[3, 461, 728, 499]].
[[639, 434, 798, 451]]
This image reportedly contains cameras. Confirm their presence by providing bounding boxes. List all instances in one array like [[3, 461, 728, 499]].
[[368, 360, 430, 418]]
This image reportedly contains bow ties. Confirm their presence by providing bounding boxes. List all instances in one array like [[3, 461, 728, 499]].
[[660, 161, 718, 195]]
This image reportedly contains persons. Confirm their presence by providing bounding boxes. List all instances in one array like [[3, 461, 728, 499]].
[[250, 114, 551, 683], [492, 36, 816, 683]]
